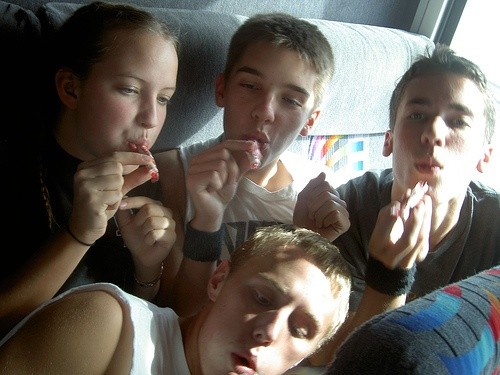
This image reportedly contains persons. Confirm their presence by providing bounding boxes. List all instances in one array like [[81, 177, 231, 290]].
[[0, 0, 178, 338], [144, 12, 352, 334], [307, 42, 500, 366], [0, 222, 354, 375]]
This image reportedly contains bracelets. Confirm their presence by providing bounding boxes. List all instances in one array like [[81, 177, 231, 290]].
[[132, 263, 164, 287], [66, 224, 95, 246]]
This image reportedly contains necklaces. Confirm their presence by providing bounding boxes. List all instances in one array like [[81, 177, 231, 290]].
[[37, 159, 61, 232]]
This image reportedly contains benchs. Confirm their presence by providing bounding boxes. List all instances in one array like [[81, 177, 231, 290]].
[[0, 3, 437, 171]]
